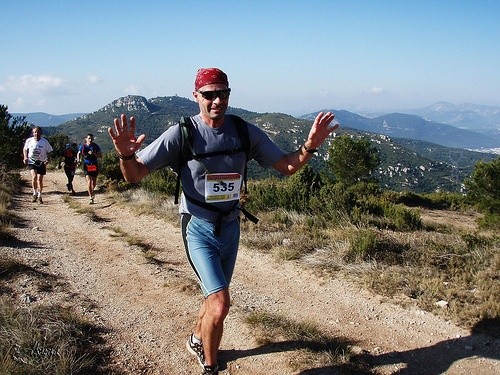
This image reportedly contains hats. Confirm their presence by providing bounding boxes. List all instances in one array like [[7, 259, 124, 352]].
[[66, 144, 72, 149], [195, 68, 229, 93]]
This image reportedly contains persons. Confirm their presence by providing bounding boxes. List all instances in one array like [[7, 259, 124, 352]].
[[23, 126, 53, 204], [58, 134, 102, 204], [107, 68, 338, 375]]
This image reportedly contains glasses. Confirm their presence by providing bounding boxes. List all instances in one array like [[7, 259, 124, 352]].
[[195, 89, 230, 100]]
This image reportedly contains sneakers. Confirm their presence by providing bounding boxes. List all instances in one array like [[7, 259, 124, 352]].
[[186, 334, 206, 368], [201, 361, 219, 375]]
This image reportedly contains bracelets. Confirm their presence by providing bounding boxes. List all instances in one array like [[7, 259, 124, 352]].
[[120, 153, 136, 160], [303, 143, 317, 154]]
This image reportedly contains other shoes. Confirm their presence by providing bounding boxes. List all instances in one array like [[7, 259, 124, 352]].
[[91, 191, 95, 197], [31, 195, 36, 202], [72, 191, 76, 195], [89, 198, 94, 204], [66, 184, 70, 191], [38, 197, 43, 205]]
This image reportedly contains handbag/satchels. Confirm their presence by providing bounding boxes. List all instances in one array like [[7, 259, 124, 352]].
[[35, 160, 42, 166]]
